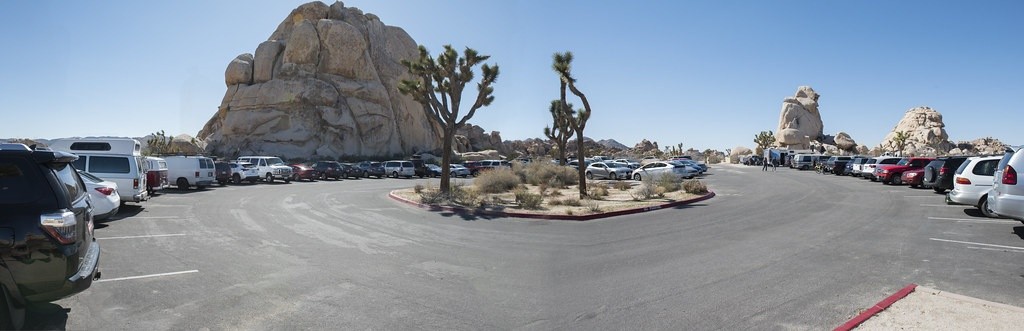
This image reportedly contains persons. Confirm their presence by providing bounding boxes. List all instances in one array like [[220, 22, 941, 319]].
[[761, 157, 767, 171], [772, 157, 777, 172]]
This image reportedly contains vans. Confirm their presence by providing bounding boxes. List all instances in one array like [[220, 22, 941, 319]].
[[48, 135, 151, 207], [160, 152, 216, 191]]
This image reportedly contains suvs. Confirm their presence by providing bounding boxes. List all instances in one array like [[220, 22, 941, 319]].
[[874, 157, 935, 186], [0, 145, 103, 331], [861, 155, 903, 182], [985, 142, 1024, 225], [947, 156, 1006, 219], [922, 156, 968, 194]]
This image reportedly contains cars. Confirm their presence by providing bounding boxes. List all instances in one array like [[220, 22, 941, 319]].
[[77, 168, 122, 224], [464, 154, 708, 180], [450, 163, 470, 178], [212, 151, 428, 187], [147, 154, 171, 197], [630, 162, 682, 181], [425, 163, 442, 179], [900, 168, 924, 189], [740, 147, 870, 178], [585, 160, 634, 181]]
